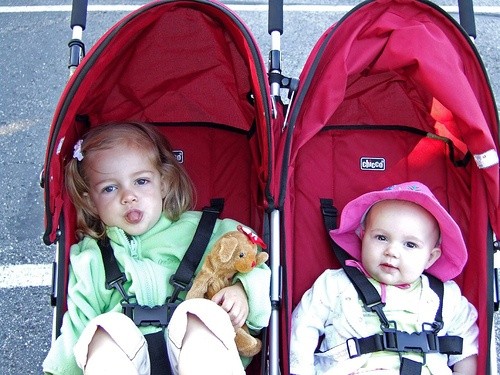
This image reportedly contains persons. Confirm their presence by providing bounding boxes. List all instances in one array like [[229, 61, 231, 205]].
[[290, 181, 479, 375], [42, 121, 271, 375]]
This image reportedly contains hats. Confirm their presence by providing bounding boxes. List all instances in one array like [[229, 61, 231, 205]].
[[329, 181, 469, 283]]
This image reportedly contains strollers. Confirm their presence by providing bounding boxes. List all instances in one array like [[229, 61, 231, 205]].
[[40, 0, 281, 375], [281, 0, 500, 375]]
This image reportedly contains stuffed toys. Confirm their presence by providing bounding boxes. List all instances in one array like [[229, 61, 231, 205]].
[[185, 225, 268, 357]]
